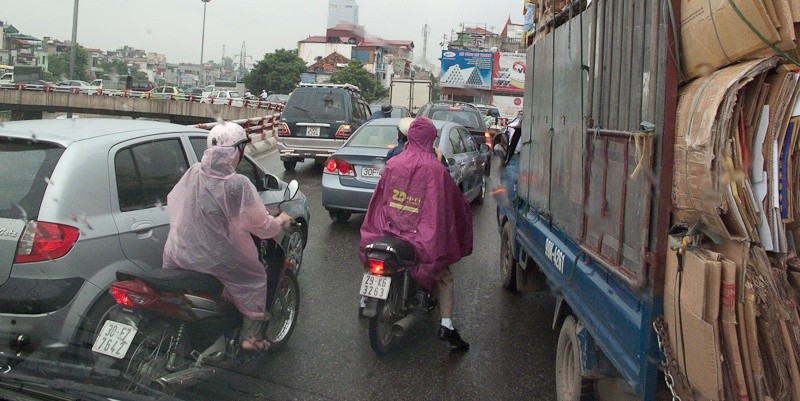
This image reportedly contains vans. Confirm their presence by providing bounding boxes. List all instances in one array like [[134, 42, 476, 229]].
[[130, 81, 156, 98], [276, 82, 372, 173]]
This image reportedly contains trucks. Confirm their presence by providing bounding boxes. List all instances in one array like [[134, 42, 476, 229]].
[[390, 77, 432, 115], [491, 0, 682, 401], [88, 74, 133, 98], [202, 80, 245, 99], [0, 64, 55, 93]]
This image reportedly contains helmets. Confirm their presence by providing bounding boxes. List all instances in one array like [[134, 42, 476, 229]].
[[398, 117, 415, 138], [207, 121, 251, 147]]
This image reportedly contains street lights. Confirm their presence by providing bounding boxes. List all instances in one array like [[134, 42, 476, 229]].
[[199, 1, 213, 86]]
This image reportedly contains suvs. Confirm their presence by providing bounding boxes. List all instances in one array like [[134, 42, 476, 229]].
[[417, 101, 492, 176], [470, 103, 502, 120], [207, 89, 243, 107]]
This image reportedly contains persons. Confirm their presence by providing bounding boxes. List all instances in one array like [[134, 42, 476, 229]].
[[242, 88, 251, 102], [164, 121, 294, 350], [359, 117, 474, 348], [258, 89, 267, 101], [384, 117, 450, 176]]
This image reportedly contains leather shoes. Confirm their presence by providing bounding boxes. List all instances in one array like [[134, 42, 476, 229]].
[[437, 326, 470, 349]]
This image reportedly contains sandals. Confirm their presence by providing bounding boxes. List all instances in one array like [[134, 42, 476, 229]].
[[240, 337, 277, 352]]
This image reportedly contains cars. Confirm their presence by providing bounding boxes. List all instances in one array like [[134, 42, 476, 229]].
[[366, 105, 412, 119], [53, 80, 91, 95], [0, 118, 310, 342], [189, 88, 204, 102], [324, 118, 487, 223], [266, 94, 290, 107], [140, 85, 186, 101]]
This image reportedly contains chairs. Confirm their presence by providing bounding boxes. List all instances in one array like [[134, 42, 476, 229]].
[[368, 135, 382, 145]]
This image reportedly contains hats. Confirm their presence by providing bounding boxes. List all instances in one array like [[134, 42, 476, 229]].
[[435, 130, 440, 137]]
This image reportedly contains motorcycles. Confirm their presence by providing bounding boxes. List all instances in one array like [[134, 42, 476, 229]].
[[106, 179, 300, 395], [360, 235, 438, 356]]
